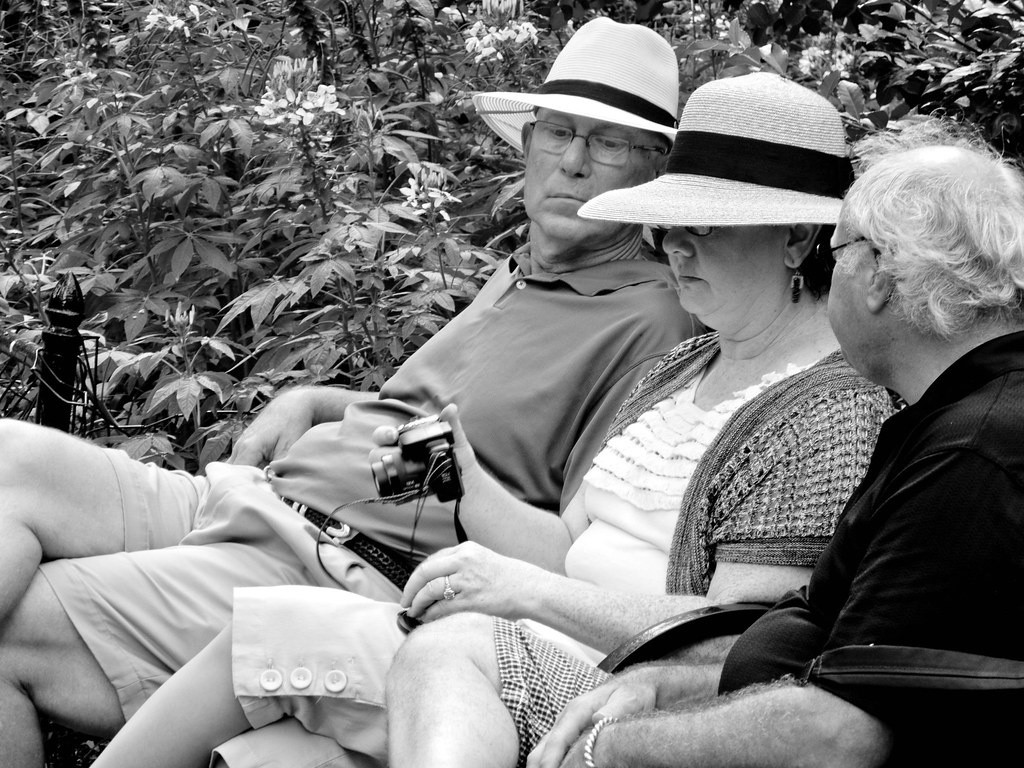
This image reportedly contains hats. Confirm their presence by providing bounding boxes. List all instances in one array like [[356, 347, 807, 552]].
[[472, 17, 678, 154], [578, 72, 856, 227]]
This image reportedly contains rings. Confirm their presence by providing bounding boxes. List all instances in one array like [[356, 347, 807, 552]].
[[444, 576, 455, 599]]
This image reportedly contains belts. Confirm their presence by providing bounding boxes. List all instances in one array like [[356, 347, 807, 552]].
[[281, 496, 417, 592]]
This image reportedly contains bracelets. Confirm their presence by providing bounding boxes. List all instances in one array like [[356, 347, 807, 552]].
[[584, 717, 619, 768]]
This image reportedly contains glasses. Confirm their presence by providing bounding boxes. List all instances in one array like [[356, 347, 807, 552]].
[[658, 225, 720, 237], [817, 236, 881, 266], [530, 120, 665, 167]]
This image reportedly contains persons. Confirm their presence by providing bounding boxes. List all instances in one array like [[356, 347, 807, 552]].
[[92, 73, 890, 768], [0, 17, 704, 768], [385, 120, 1023, 768]]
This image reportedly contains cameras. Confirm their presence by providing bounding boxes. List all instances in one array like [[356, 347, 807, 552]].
[[371, 414, 465, 505]]
